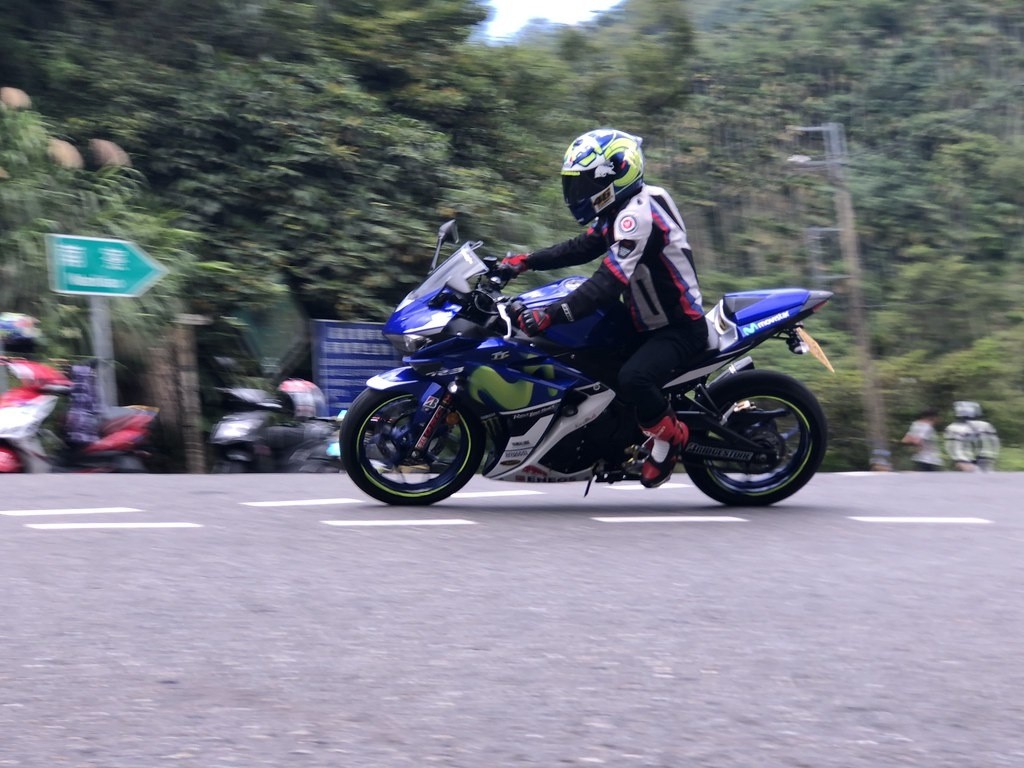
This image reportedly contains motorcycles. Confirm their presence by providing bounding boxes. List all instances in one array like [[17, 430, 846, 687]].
[[338, 219, 835, 508], [204, 384, 343, 473], [0, 350, 161, 476]]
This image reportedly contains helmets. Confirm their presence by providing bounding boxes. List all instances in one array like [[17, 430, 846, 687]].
[[560, 130, 644, 227], [0, 312, 47, 354]]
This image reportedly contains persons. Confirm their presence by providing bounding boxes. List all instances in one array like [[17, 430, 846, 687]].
[[945, 402, 1000, 473], [492, 129, 709, 488], [902, 406, 945, 471]]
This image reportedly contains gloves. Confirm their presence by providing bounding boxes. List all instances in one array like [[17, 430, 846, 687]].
[[502, 251, 527, 276], [518, 308, 552, 337]]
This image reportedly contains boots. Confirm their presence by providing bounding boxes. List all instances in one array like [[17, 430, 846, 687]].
[[638, 402, 689, 488]]
